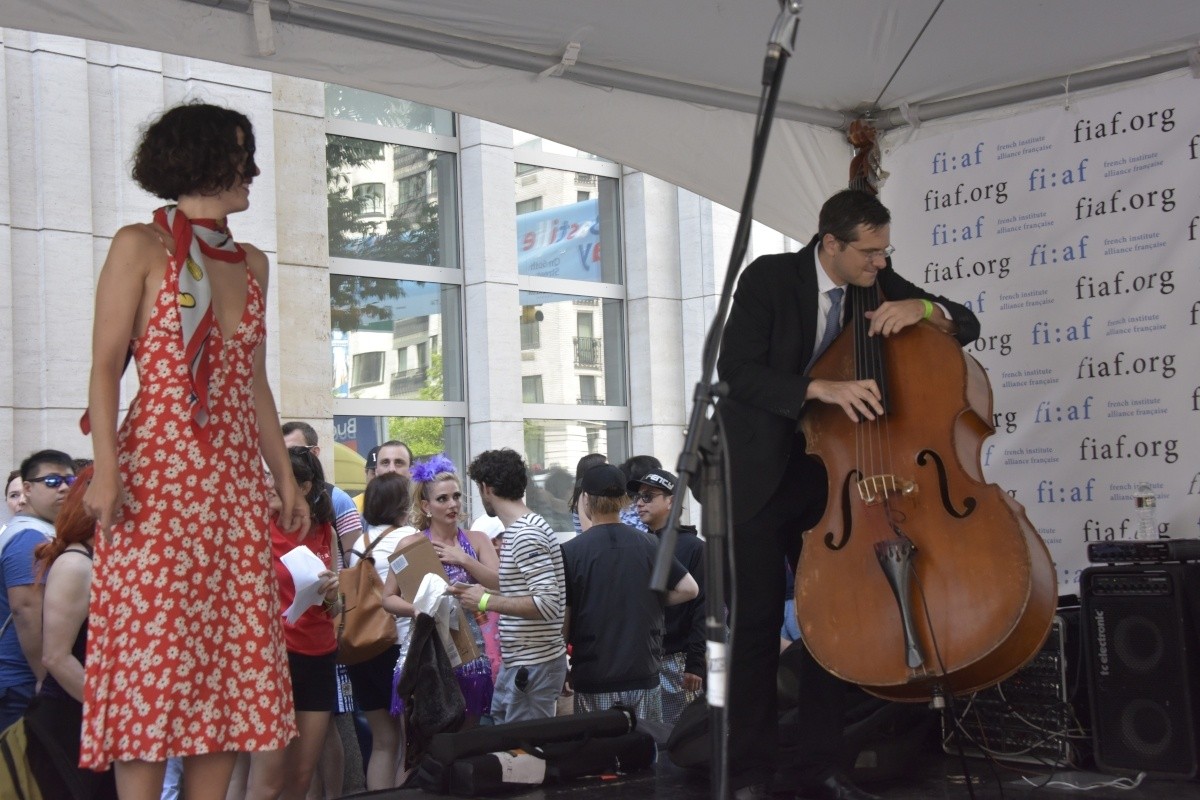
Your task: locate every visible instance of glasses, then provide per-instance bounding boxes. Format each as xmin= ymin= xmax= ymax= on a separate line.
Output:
xmin=838 ymin=238 xmax=896 ymax=259
xmin=27 ymin=474 xmax=79 ymax=488
xmin=631 ymin=492 xmax=671 ymax=503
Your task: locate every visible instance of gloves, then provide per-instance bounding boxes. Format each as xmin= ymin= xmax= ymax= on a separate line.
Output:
xmin=581 ymin=463 xmax=628 ymax=497
xmin=626 ymin=469 xmax=679 ymax=498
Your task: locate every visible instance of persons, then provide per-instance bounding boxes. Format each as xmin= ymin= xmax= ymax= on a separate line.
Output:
xmin=677 ymin=191 xmax=983 ymax=800
xmin=77 ymin=100 xmax=314 ymax=800
xmin=0 ymin=449 xmax=182 ymax=800
xmin=226 ymin=421 xmax=803 ymax=800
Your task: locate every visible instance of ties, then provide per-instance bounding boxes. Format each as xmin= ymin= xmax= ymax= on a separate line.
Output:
xmin=800 ymin=288 xmax=844 ymax=376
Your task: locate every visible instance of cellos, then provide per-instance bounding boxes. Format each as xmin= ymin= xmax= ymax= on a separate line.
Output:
xmin=795 ymin=121 xmax=1058 ymax=800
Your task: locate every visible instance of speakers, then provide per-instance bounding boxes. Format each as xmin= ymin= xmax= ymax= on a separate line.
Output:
xmin=1079 ymin=561 xmax=1200 ymax=778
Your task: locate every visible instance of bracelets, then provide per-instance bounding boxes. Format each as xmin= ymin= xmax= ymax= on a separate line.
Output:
xmin=922 ymin=298 xmax=935 ymax=318
xmin=324 ymin=593 xmax=339 ymax=606
xmin=479 ymin=593 xmax=491 ymax=611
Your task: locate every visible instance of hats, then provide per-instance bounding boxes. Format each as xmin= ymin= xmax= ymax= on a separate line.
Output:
xmin=366 ymin=446 xmax=381 ymax=468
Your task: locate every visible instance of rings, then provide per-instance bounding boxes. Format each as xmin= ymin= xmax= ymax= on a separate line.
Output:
xmin=439 ymin=552 xmax=441 ymax=556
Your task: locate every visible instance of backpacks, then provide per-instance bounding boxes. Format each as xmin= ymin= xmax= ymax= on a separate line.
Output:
xmin=334 ymin=525 xmax=406 ymax=665
